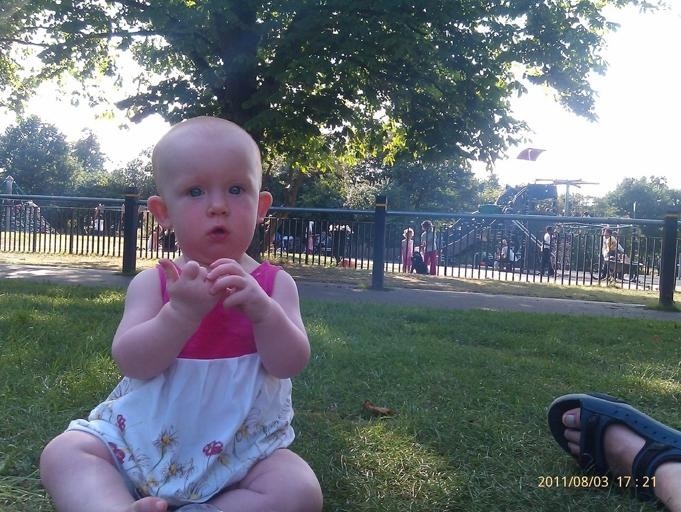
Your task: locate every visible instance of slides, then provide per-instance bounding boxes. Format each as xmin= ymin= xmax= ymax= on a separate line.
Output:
xmin=438 ymin=184 xmax=557 ymax=257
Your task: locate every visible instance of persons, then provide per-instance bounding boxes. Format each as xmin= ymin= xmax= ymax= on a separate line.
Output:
xmin=401 ymin=228 xmax=414 ymax=273
xmin=40 ymin=115 xmax=325 ymax=512
xmin=582 ymin=211 xmax=591 ymax=218
xmin=494 ymin=226 xmax=625 ymax=280
xmin=420 ymin=220 xmax=437 ymax=275
xmin=147 ymin=221 xmax=163 ymax=251
xmin=259 ymin=219 xmax=352 ymax=263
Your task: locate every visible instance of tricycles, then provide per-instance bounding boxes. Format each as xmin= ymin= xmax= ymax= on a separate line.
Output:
xmin=590 ymin=249 xmax=644 ymax=282
